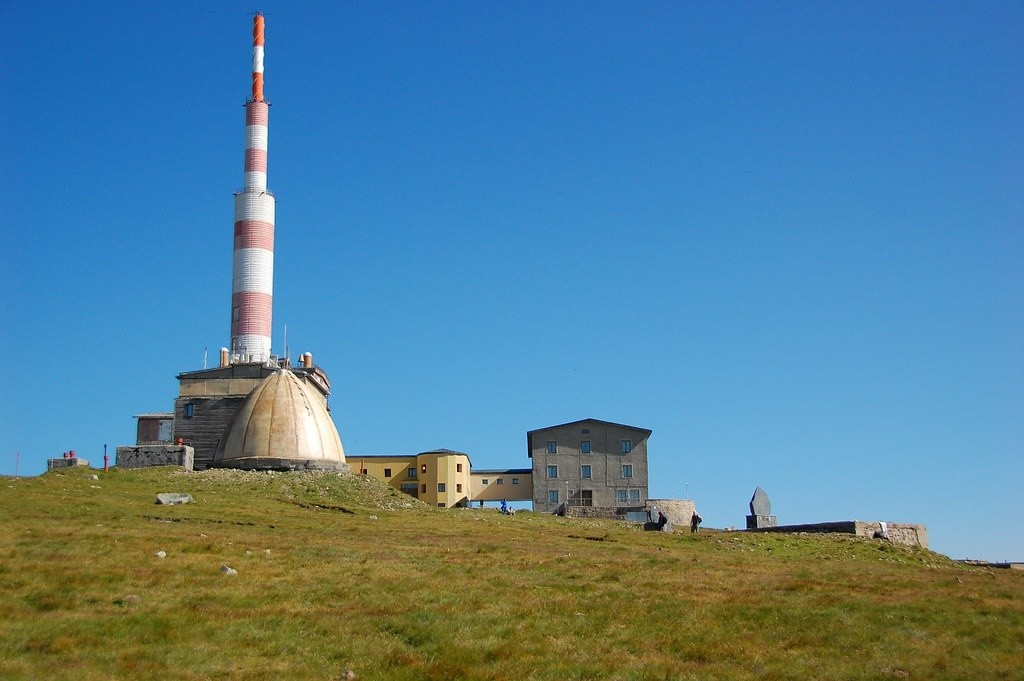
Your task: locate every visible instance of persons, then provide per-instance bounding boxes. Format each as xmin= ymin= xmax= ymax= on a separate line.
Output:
xmin=657 ymin=511 xmax=668 ymax=531
xmin=690 ymin=511 xmax=703 ymax=533
xmin=500 ymin=497 xmax=508 ymax=515
xmin=507 ymin=507 xmax=515 ymax=516
